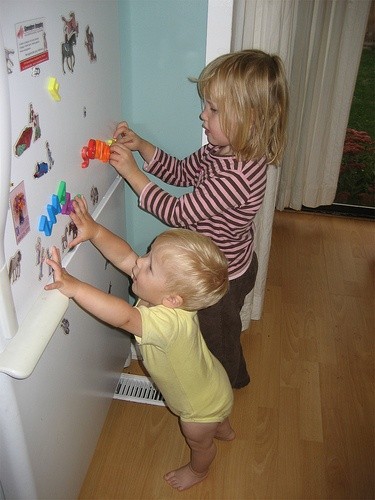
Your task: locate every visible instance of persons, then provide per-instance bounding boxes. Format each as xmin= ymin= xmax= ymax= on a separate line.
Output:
xmin=105 ymin=49 xmax=288 ymax=388
xmin=46 ymin=195 xmax=236 ymax=494
